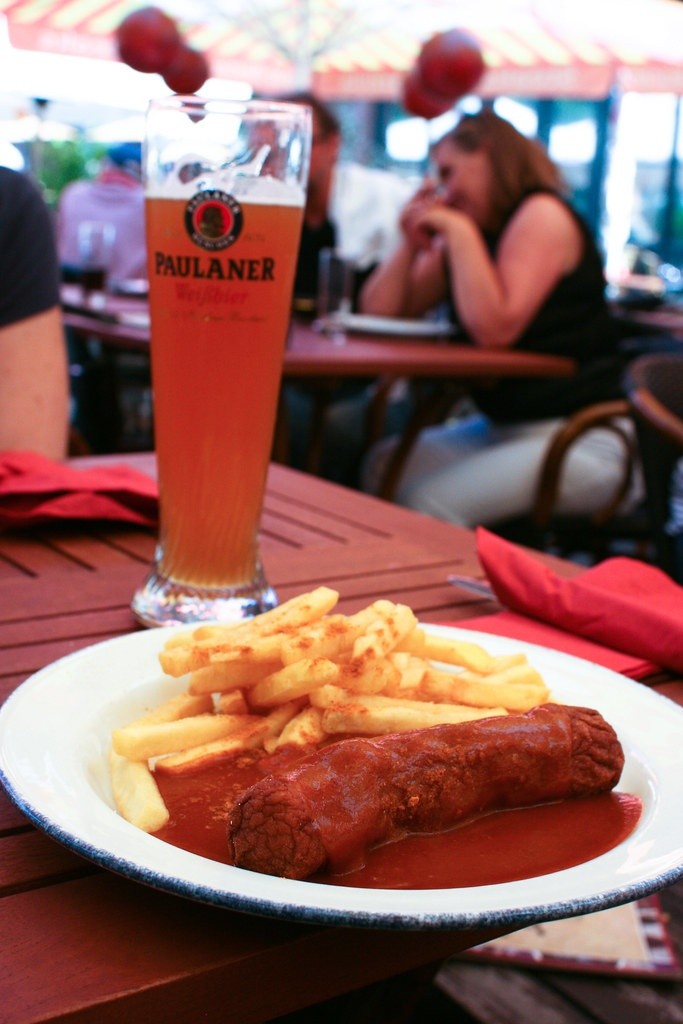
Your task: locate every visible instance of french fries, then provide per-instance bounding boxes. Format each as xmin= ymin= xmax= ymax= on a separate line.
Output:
xmin=109 ymin=585 xmax=557 ymax=834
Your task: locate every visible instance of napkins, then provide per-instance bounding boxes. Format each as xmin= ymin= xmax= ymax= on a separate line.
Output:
xmin=464 ymin=521 xmax=682 ymax=699
xmin=0 ymin=451 xmax=165 ymax=532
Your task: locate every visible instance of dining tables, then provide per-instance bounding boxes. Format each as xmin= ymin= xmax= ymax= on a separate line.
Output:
xmin=0 ymin=383 xmax=683 ymax=1023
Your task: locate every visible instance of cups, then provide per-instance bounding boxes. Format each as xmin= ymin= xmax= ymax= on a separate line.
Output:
xmin=130 ymin=96 xmax=312 ymax=629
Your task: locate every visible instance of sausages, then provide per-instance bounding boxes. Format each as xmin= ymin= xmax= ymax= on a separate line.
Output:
xmin=227 ymin=706 xmax=624 ymax=883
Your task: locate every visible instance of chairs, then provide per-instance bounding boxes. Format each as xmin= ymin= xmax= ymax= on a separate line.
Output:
xmin=515 ymin=351 xmax=683 ymax=591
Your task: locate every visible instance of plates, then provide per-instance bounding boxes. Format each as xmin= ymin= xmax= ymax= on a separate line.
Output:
xmin=1 ymin=622 xmax=683 ymax=924
xmin=333 ymin=311 xmax=449 ymax=334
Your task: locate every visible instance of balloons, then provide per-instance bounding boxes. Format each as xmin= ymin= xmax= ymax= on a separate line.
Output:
xmin=405 ymin=31 xmax=482 ymax=115
xmin=121 ymin=8 xmax=205 ymax=95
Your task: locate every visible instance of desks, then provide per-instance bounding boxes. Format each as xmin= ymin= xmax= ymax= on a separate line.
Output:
xmin=53 ymin=295 xmax=580 ymax=505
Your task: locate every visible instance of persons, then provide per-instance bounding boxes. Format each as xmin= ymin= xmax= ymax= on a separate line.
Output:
xmin=362 ymin=113 xmax=635 ymax=525
xmin=0 ymin=165 xmax=70 ymax=464
xmin=250 ymin=93 xmax=426 ymax=476
xmin=57 ymin=142 xmax=150 ymax=287
